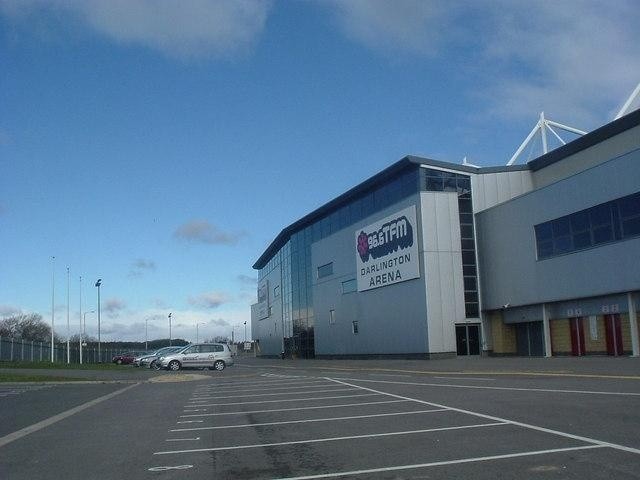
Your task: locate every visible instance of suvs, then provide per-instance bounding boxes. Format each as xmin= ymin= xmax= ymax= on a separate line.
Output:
xmin=112 ymin=343 xmax=234 ymax=371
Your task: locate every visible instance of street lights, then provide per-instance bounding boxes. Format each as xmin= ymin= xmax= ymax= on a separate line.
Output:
xmin=243 ymin=321 xmax=248 ymax=342
xmin=94 ymin=278 xmax=102 ymax=364
xmin=167 ymin=312 xmax=173 ymax=347
